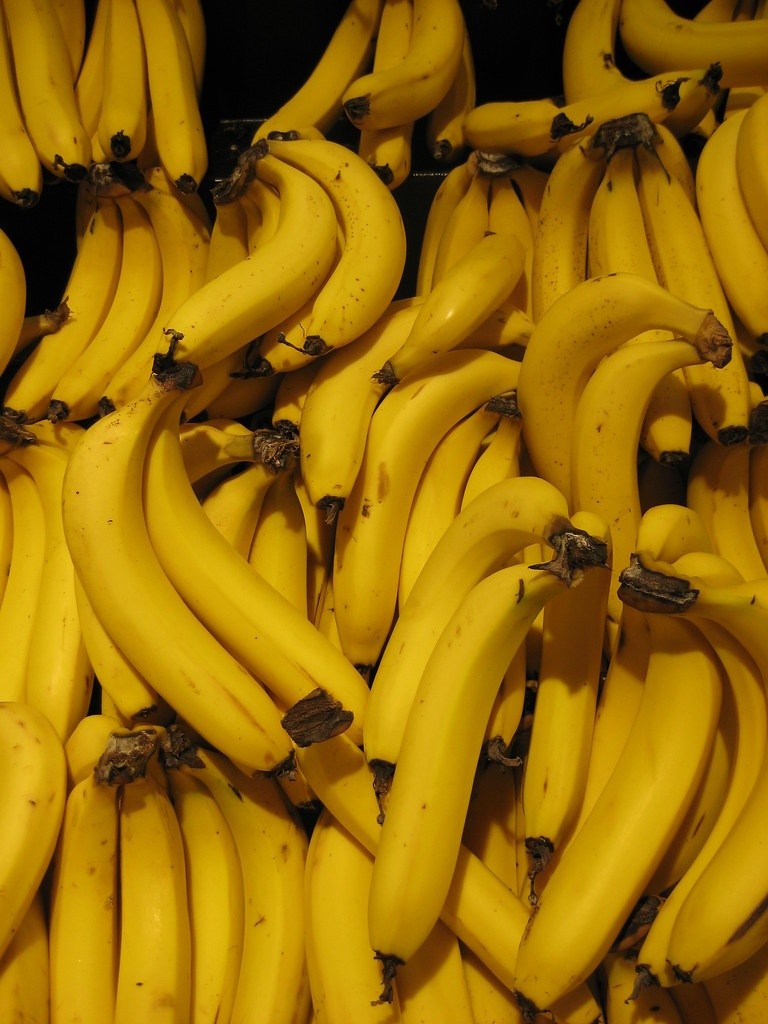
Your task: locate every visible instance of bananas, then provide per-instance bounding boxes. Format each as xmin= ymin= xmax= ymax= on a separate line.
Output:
xmin=0 ymin=0 xmax=768 ymax=1024
